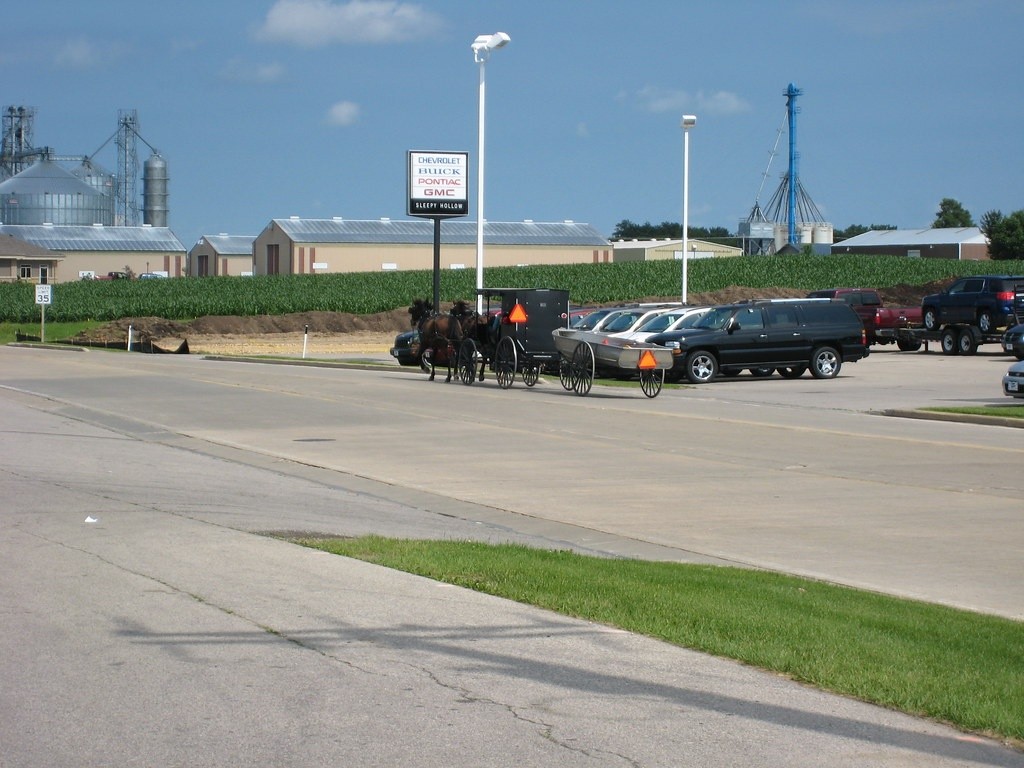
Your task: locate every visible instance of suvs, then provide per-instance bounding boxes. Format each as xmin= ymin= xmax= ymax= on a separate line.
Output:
xmin=921 ymin=273 xmax=1024 ymax=329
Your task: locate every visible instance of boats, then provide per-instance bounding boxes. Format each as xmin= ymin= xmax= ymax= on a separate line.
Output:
xmin=551 ymin=325 xmax=674 ymax=372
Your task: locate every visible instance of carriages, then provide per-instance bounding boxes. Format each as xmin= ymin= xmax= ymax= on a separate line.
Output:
xmin=407 ymin=285 xmax=570 ymax=387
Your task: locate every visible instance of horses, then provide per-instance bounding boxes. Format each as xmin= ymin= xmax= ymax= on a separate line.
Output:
xmin=408 ymin=297 xmax=509 ymax=383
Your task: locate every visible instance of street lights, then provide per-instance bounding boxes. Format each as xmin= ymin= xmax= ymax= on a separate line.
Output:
xmin=680 ymin=113 xmax=697 ymax=305
xmin=470 ymin=32 xmax=510 ymax=314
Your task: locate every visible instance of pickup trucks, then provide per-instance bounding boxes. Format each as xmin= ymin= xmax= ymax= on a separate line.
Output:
xmin=95 ymin=272 xmax=127 ymax=281
xmin=646 ymin=297 xmax=870 ymax=381
xmin=807 ymin=289 xmax=927 ymax=351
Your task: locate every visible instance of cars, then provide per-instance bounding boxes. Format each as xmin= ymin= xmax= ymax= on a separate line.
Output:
xmin=570 ymin=297 xmax=709 ymax=378
xmin=139 ymin=273 xmax=161 ymax=278
xmin=1001 ymin=323 xmax=1024 ymax=355
xmin=390 ymin=327 xmax=485 ymax=367
xmin=1002 ymin=362 xmax=1024 ymax=398
